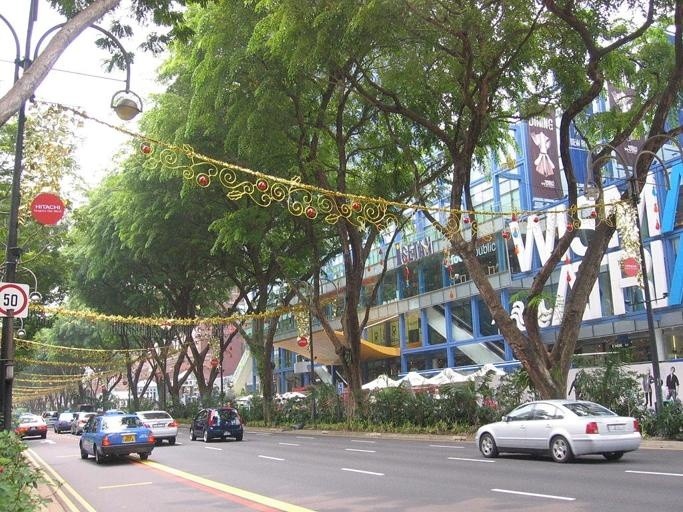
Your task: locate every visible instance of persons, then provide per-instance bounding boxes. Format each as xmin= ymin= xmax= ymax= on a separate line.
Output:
xmin=665 ymin=367 xmax=679 ymax=403
xmin=568 ymin=373 xmax=583 ymax=400
xmin=276 ymin=394 xmax=307 ymax=420
xmin=478 ymin=370 xmax=499 ymax=409
xmin=637 ymin=368 xmax=654 ymax=408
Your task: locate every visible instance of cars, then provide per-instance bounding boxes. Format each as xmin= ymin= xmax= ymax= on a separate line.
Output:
xmin=132 ymin=410 xmax=178 ymax=446
xmin=188 ymin=406 xmax=243 ymax=443
xmin=474 ymin=399 xmax=642 ymax=463
xmin=10 ymin=403 xmax=125 ymax=440
xmin=78 ymin=409 xmax=155 ymax=463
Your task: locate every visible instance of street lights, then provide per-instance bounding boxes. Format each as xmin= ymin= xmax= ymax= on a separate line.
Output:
xmin=583 ymin=134 xmax=682 ymax=438
xmin=0 ymin=255 xmax=43 ymax=339
xmin=45 ymin=367 xmax=175 ymax=411
xmin=277 ymin=277 xmax=340 ymax=424
xmin=194 ymin=308 xmax=247 ymax=407
xmin=0 ymin=1 xmax=143 ymax=446
